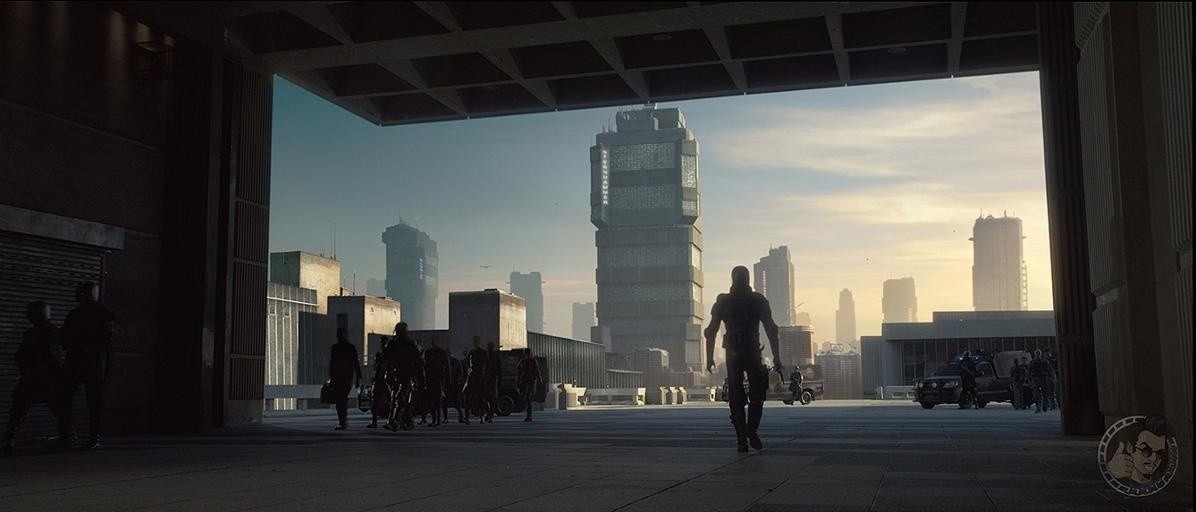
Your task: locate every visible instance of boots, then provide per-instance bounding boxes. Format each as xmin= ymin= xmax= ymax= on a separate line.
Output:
xmin=1035 ymin=403 xmax=1056 ymax=413
xmin=480 ymin=409 xmax=494 ymax=424
xmin=733 ymin=404 xmax=762 ymax=451
xmin=367 ymin=407 xmax=448 ymax=432
xmin=458 ymin=408 xmax=471 ymax=424
xmin=335 ymin=409 xmax=347 ymax=429
xmin=524 ymin=407 xmax=531 ymax=422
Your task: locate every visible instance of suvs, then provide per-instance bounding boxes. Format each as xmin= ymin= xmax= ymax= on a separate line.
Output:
xmin=352 ymin=346 xmax=550 ymax=424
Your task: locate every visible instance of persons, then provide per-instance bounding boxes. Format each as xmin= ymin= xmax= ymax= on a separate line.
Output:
xmin=788 ymin=365 xmax=805 ymax=405
xmin=52 ymin=280 xmax=120 ymax=450
xmin=948 ymin=349 xmax=983 ymax=410
xmin=1 ymin=299 xmax=64 ymax=451
xmin=363 ymin=321 xmax=539 ymax=431
xmin=705 ymin=264 xmax=783 ymax=453
xmin=1010 ymin=346 xmax=1060 ymax=413
xmin=1107 ymin=415 xmax=1171 ymax=487
xmin=319 ymin=325 xmax=361 ymax=430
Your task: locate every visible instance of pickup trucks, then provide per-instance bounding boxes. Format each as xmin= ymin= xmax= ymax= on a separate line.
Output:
xmin=910 ymin=350 xmax=1043 ymax=410
xmin=720 ymin=366 xmax=825 ymax=407
